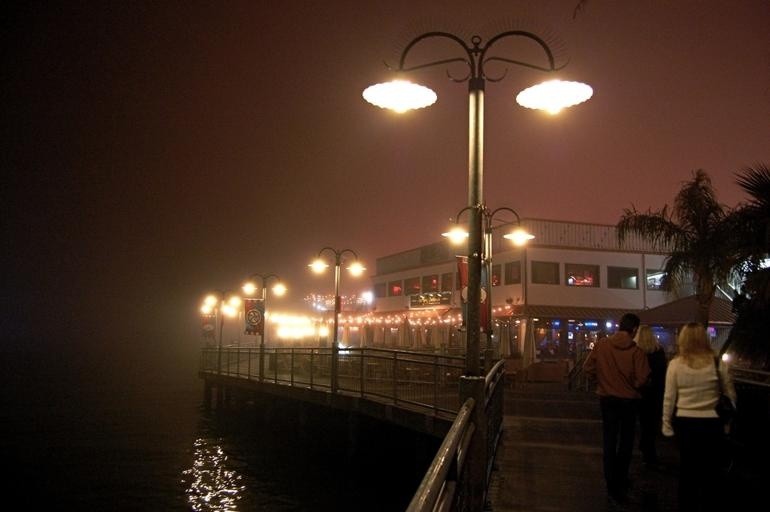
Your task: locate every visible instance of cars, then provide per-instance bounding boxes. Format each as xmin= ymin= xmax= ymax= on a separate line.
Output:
xmin=720 ymin=337 xmax=770 ymax=423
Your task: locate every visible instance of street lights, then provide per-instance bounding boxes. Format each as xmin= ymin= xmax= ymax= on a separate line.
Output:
xmin=242 ymin=272 xmax=286 ymax=383
xmin=360 ymin=30 xmax=595 ymax=397
xmin=442 ymin=202 xmax=537 ymax=376
xmin=307 ymin=245 xmax=368 ymax=397
xmin=198 ymin=291 xmax=242 ymax=375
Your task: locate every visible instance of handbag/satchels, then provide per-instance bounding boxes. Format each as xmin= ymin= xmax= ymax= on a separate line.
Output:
xmin=712 ymin=396 xmax=735 ymax=421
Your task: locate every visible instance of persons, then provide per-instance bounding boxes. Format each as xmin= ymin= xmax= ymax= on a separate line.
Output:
xmin=579 ymin=313 xmax=651 ymax=503
xmin=634 ymin=325 xmax=667 ymax=461
xmin=661 ymin=322 xmax=736 ymax=512
